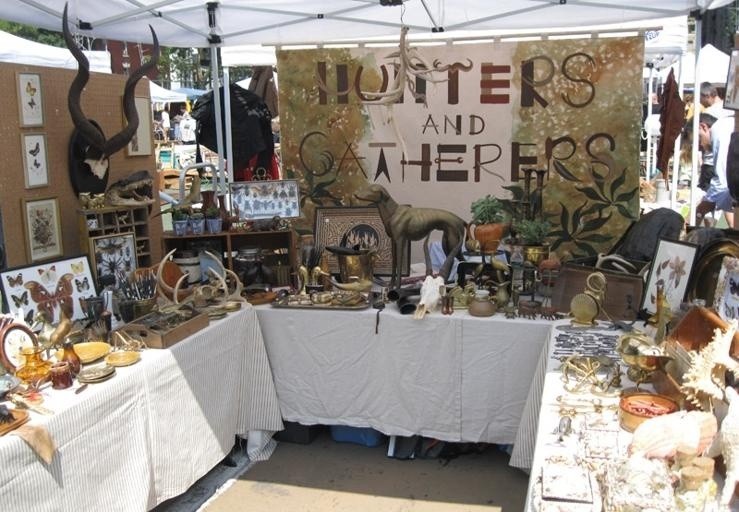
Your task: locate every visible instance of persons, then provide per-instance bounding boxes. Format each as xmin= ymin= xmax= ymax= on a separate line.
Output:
xmin=699 ymin=82 xmax=735 ymax=118
xmin=157 ymin=107 xmax=170 ymax=143
xmin=179 ymin=105 xmax=190 ymax=121
xmin=684 ymin=94 xmax=706 ymax=121
xmin=683 ymin=113 xmax=735 ymax=228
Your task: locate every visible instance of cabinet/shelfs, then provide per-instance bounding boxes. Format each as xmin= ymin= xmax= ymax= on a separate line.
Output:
xmin=75 ymin=205 xmax=154 ymax=269
xmin=161 ymin=230 xmax=298 ymax=294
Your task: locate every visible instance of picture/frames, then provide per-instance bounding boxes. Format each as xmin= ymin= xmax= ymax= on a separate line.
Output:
xmin=229 ymin=180 xmax=301 ymax=221
xmin=639 ymin=235 xmax=739 ymax=327
xmin=313 ymin=204 xmax=411 ymax=276
xmin=0 ymin=232 xmax=138 ymax=333
xmin=14 ymin=71 xmax=63 ymax=264
xmin=122 ymin=96 xmax=155 ymax=157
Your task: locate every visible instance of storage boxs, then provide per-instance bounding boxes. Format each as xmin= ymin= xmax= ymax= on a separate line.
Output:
xmin=272 ymin=421 xmax=321 ymax=445
xmin=330 ymin=424 xmax=388 ymax=447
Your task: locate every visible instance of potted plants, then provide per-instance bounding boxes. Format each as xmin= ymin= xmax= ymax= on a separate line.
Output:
xmin=170 ymin=207 xmax=223 ymax=237
xmin=471 ymin=194 xmax=550 ymax=267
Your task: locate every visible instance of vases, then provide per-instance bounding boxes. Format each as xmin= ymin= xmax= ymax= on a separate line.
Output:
xmin=201 ymin=191 xmax=229 ymax=230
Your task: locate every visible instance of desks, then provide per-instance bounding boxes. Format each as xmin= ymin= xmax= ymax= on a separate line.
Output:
xmin=1 ymin=285 xmax=286 ymax=511
xmin=250 ymin=286 xmax=568 ymax=460
xmin=509 ymin=317 xmax=738 ymax=510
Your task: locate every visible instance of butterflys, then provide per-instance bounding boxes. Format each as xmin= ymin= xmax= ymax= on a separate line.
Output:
xmin=25 ymin=80 xmax=37 ymax=108
xmin=28 ymin=142 xmax=41 ymax=169
xmin=6 ymin=260 xmax=94 ymax=329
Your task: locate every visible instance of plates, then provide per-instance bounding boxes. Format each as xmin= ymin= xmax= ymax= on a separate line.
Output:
xmin=53 ymin=341 xmax=140 ymax=384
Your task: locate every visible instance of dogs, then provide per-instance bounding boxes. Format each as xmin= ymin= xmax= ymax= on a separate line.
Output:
xmin=354 ymin=183 xmax=471 ymax=290
